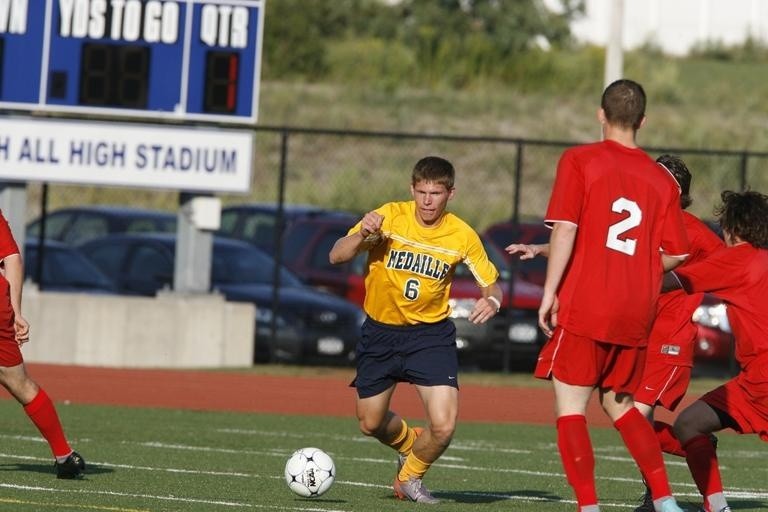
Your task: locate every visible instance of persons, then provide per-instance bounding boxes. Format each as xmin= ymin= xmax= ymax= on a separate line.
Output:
xmin=505 ymin=155 xmax=724 ymax=512
xmin=328 ymin=156 xmax=504 ymax=505
xmin=654 ymin=186 xmax=768 ymax=511
xmin=533 ymin=79 xmax=692 ymax=512
xmin=0 ymin=210 xmax=86 ymax=478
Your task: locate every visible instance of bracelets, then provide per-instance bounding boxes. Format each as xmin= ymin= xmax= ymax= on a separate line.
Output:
xmin=488 ymin=295 xmax=502 ymax=314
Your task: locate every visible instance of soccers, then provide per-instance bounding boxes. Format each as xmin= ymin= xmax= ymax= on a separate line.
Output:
xmin=283 ymin=446 xmax=337 ymax=498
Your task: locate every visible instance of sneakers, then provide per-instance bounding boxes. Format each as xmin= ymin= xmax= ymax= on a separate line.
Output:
xmin=53 ymin=451 xmax=86 ymax=481
xmin=633 ymin=485 xmax=656 ymax=512
xmin=394 ymin=474 xmax=441 ymax=504
xmin=708 ymin=432 xmax=718 ymax=452
xmin=393 ymin=427 xmax=425 ymax=500
